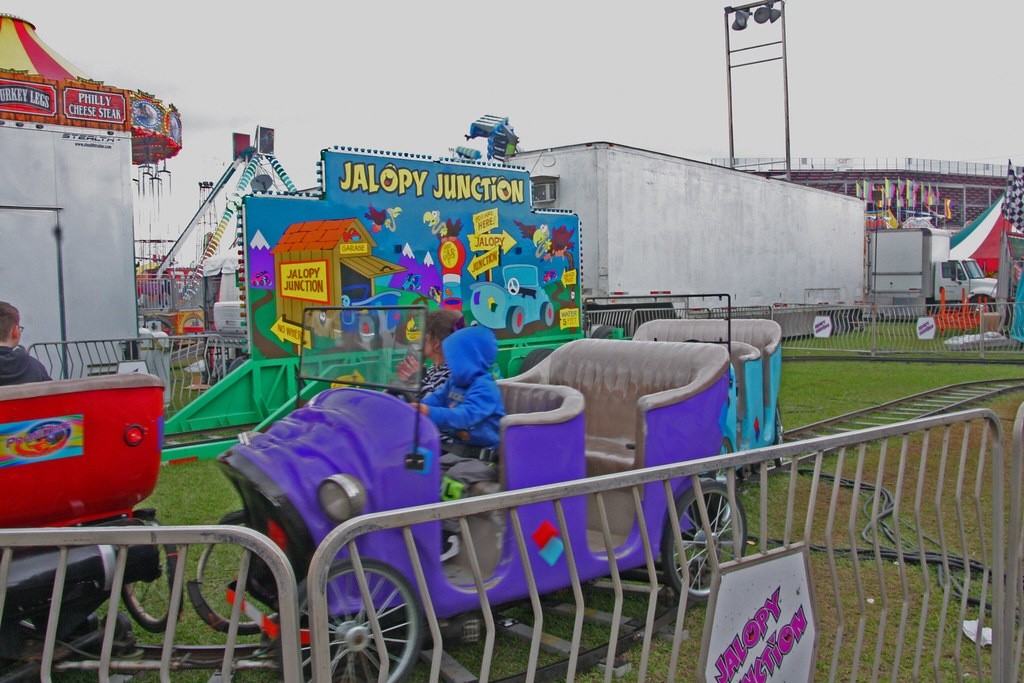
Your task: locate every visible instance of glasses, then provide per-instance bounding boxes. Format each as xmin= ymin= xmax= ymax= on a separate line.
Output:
xmin=19 ymin=326 xmax=23 ymax=332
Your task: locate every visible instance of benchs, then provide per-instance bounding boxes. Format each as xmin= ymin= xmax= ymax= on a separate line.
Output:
xmin=585 ymin=436 xmax=640 ymax=493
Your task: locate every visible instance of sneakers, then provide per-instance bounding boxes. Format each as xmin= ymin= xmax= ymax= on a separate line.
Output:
xmin=440 ymin=538 xmax=461 ymax=564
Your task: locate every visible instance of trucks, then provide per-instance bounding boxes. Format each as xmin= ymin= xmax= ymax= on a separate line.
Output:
xmin=869 ymin=228 xmax=1014 ymax=326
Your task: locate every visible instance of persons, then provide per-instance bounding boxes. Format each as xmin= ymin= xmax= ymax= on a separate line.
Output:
xmin=407 ymin=326 xmax=507 ymax=563
xmin=397 ymin=308 xmax=468 ymax=459
xmin=0 ymin=301 xmax=53 ymax=387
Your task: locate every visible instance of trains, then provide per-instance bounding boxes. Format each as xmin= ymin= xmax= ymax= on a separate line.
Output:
xmin=0 ymin=286 xmax=788 ymax=683
